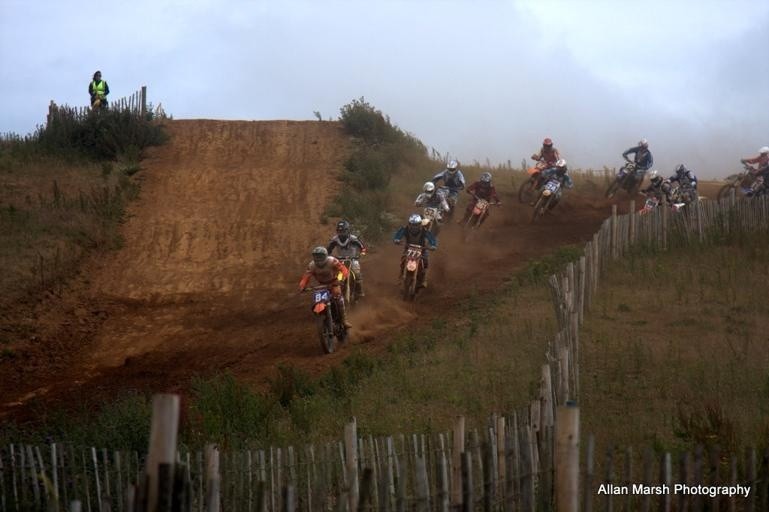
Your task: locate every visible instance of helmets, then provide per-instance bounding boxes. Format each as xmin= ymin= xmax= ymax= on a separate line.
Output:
xmin=676 ymin=163 xmax=687 ymax=175
xmin=648 ymin=170 xmax=662 ymax=186
xmin=639 ymin=138 xmax=648 ymax=149
xmin=480 ymin=172 xmax=492 ymax=182
xmin=408 ymin=214 xmax=422 ymax=232
xmin=543 ymin=137 xmax=553 ymax=145
xmin=556 ymin=159 xmax=567 ymax=175
xmin=423 ymin=182 xmax=435 ymax=200
xmin=759 ymin=147 xmax=769 ymax=153
xmin=312 ymin=247 xmax=329 ymax=268
xmin=337 ymin=221 xmax=349 ymax=238
xmin=447 ymin=161 xmax=458 ymax=174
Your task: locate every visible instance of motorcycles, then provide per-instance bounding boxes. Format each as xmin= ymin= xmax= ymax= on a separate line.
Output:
xmin=332 ymin=253 xmax=366 ymax=304
xmin=433 ymin=182 xmax=461 ymax=221
xmin=300 ymin=277 xmax=348 ymax=357
xmin=415 ymin=203 xmax=444 ymax=234
xmin=603 ymin=150 xmax=769 ymax=225
xmin=396 ymin=241 xmax=432 ymax=303
xmin=517 ymin=155 xmax=553 ymax=203
xmin=527 ymin=174 xmax=567 ymax=223
xmin=461 ymin=189 xmax=496 ymax=239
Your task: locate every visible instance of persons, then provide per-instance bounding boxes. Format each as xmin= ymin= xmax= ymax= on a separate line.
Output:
xmin=530 ymin=158 xmax=572 ymax=216
xmin=415 ymin=182 xmax=450 ymax=236
xmin=89 ymin=70 xmax=109 ymax=114
xmin=456 ymin=172 xmax=503 ymax=231
xmin=530 ymin=138 xmax=560 ymax=167
xmin=431 ymin=161 xmax=465 ymax=214
xmin=327 ymin=220 xmax=366 ymax=297
xmin=623 ymin=139 xmax=769 ymax=207
xmin=297 ymin=247 xmax=352 ymax=329
xmin=393 ymin=215 xmax=436 ymax=287
